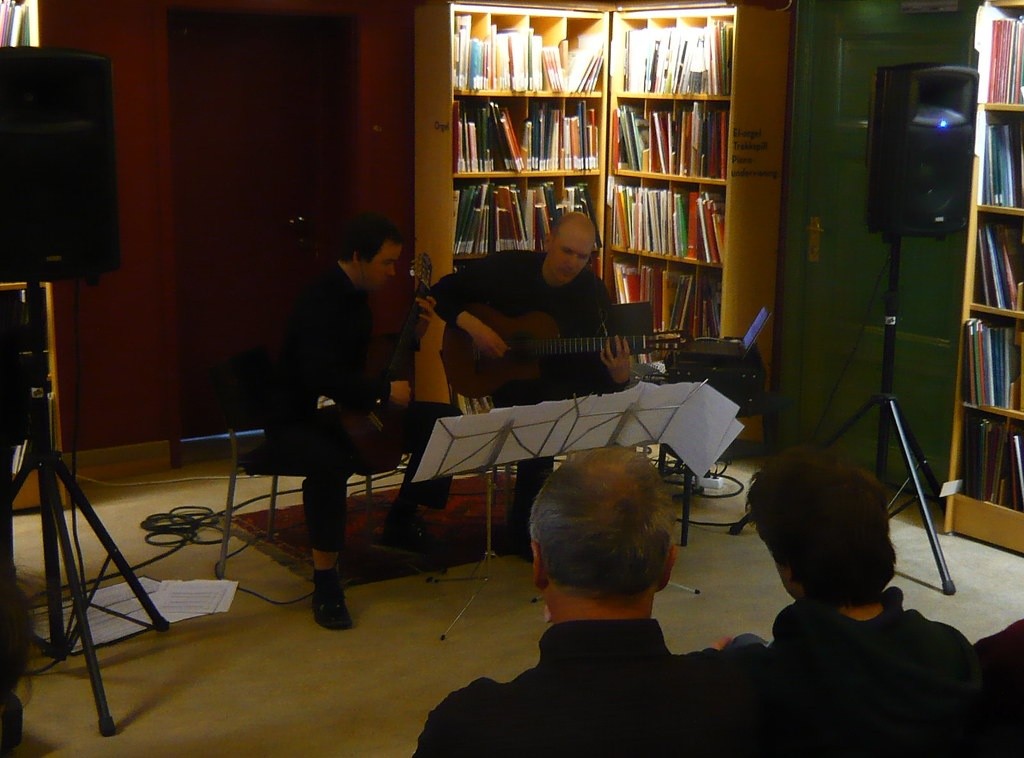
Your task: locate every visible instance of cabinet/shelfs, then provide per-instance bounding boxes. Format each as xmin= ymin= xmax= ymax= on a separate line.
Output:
xmin=414 ymin=2 xmax=791 ymax=442
xmin=0 ymin=0 xmax=72 ymax=511
xmin=943 ymin=0 xmax=1024 ymax=558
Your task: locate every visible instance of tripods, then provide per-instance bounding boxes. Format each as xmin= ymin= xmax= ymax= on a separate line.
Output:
xmin=2 ymin=282 xmax=171 ymax=738
xmin=730 ymin=240 xmax=956 ymax=595
xmin=413 ymin=391 xmax=594 ymax=640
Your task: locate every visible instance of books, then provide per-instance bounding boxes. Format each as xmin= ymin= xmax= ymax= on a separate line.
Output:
xmin=453 ymin=16 xmax=732 ymax=363
xmin=958 ymin=17 xmax=1024 ymax=515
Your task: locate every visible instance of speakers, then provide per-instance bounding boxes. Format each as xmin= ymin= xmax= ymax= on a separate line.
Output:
xmin=864 ymin=62 xmax=980 ymax=238
xmin=0 ymin=46 xmax=117 ymax=290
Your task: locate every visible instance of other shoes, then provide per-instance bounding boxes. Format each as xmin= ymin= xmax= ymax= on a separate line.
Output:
xmin=385 ymin=514 xmax=441 ymax=558
xmin=510 ymin=526 xmax=535 ymax=563
xmin=313 ymin=588 xmax=352 ymax=629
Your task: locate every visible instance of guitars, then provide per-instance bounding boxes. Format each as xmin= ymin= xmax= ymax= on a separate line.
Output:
xmin=332 ymin=246 xmax=435 ymax=475
xmin=440 ymin=301 xmax=693 ymax=400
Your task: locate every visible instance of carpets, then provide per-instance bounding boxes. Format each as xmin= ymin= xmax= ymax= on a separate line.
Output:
xmin=222 ymin=471 xmax=516 ymax=591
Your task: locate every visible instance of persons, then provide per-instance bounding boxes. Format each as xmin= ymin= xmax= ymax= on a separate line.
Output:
xmin=713 ymin=451 xmax=1024 ymax=758
xmin=429 ymin=215 xmax=631 ymax=564
xmin=409 ymin=446 xmax=782 ymax=758
xmin=975 ymin=619 xmax=1023 ymax=674
xmin=265 ymin=214 xmax=465 ymax=631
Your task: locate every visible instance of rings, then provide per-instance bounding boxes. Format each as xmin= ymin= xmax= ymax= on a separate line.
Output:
xmin=609 ymin=356 xmax=615 ymax=362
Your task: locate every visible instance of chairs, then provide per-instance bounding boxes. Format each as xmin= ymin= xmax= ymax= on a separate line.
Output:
xmin=211 ymin=346 xmax=371 ymax=562
xmin=439 ymin=320 xmax=573 ymax=524
